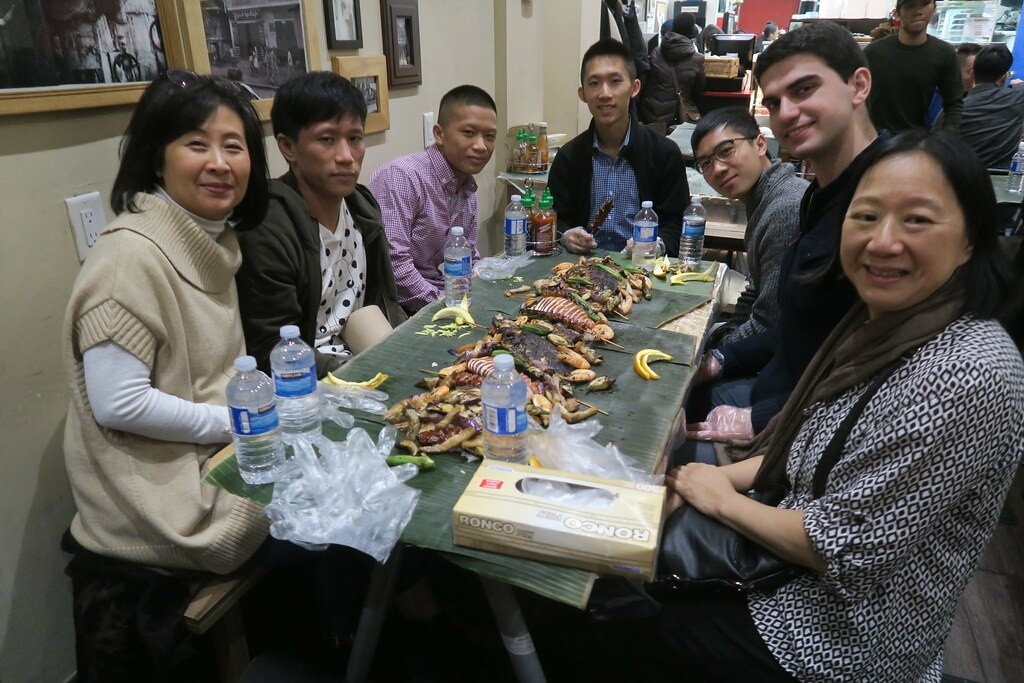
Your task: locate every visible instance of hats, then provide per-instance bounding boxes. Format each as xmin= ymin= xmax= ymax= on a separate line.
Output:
xmin=672 ymin=12 xmax=698 ymax=40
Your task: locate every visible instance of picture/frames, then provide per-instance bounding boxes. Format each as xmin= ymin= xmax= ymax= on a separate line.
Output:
xmin=379 ymin=0 xmax=422 ymax=91
xmin=322 ymin=0 xmax=364 ymax=50
xmin=0 ymin=0 xmax=323 ymax=123
xmin=331 ymin=56 xmax=390 ymax=135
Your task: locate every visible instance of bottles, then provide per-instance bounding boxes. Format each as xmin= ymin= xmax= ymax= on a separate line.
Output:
xmin=679 ymin=197 xmax=706 ymax=265
xmin=631 ymin=200 xmax=658 ymax=272
xmin=1004 ymin=142 xmax=1024 ymax=193
xmin=507 ymin=122 xmax=549 ymax=174
xmin=479 ymin=353 xmax=530 ymax=466
xmin=521 ymin=187 xmax=557 ymax=256
xmin=269 ymin=325 xmax=323 ymax=447
xmin=503 ymin=194 xmax=527 ymax=260
xmin=225 ymin=355 xmax=286 ymax=485
xmin=442 ymin=226 xmax=473 ymax=308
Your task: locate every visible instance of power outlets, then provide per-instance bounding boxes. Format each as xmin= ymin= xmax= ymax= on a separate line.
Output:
xmin=423 ymin=112 xmax=436 ymax=149
xmin=64 ymin=191 xmax=109 ymax=265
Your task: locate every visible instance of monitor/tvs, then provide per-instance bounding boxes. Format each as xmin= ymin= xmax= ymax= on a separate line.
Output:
xmin=711 ymin=33 xmax=756 ymax=77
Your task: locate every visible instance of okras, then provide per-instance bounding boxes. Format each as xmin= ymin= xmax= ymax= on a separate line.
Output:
xmin=491 ymin=310 xmax=552 ymax=368
xmin=386 ymin=454 xmax=435 ymax=470
xmin=565 ymin=263 xmax=652 ymax=322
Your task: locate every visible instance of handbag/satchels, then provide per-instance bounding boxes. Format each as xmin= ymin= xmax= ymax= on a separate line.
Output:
xmin=643 ymin=481 xmax=805 ymax=593
xmin=679 ymin=97 xmax=701 ymax=124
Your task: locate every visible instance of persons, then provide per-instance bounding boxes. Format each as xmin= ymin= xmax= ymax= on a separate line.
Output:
xmin=61 ymin=69 xmax=503 ymax=683
xmin=932 ymin=42 xmax=1024 ymax=170
xmin=666 ymin=20 xmax=891 ymax=479
xmin=248 ymin=46 xmax=298 ymax=81
xmin=357 ymin=79 xmax=370 ymax=89
xmin=861 ymin=0 xmax=963 ymax=138
xmin=53 ymin=34 xmax=140 ymax=82
xmin=235 ymin=71 xmax=412 ymax=380
xmin=636 ymin=12 xmax=727 ymax=133
xmin=514 ymin=125 xmax=1024 ymax=683
xmin=683 ymin=109 xmax=813 ymax=384
xmin=546 ymin=38 xmax=691 ymax=258
xmin=755 ymin=23 xmax=778 ymax=55
xmin=365 ymin=85 xmax=498 ymax=315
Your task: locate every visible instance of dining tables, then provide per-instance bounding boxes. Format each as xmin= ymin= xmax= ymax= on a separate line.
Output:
xmin=207 ymin=246 xmax=727 ymax=683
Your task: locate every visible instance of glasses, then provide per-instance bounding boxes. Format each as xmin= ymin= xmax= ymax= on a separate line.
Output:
xmin=149 ymin=69 xmax=242 ymax=95
xmin=694 ymin=138 xmax=755 ymax=174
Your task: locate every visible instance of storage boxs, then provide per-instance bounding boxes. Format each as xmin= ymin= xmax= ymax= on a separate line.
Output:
xmin=704 ymin=56 xmax=739 ymax=78
xmin=451 ymin=458 xmax=667 ymax=582
